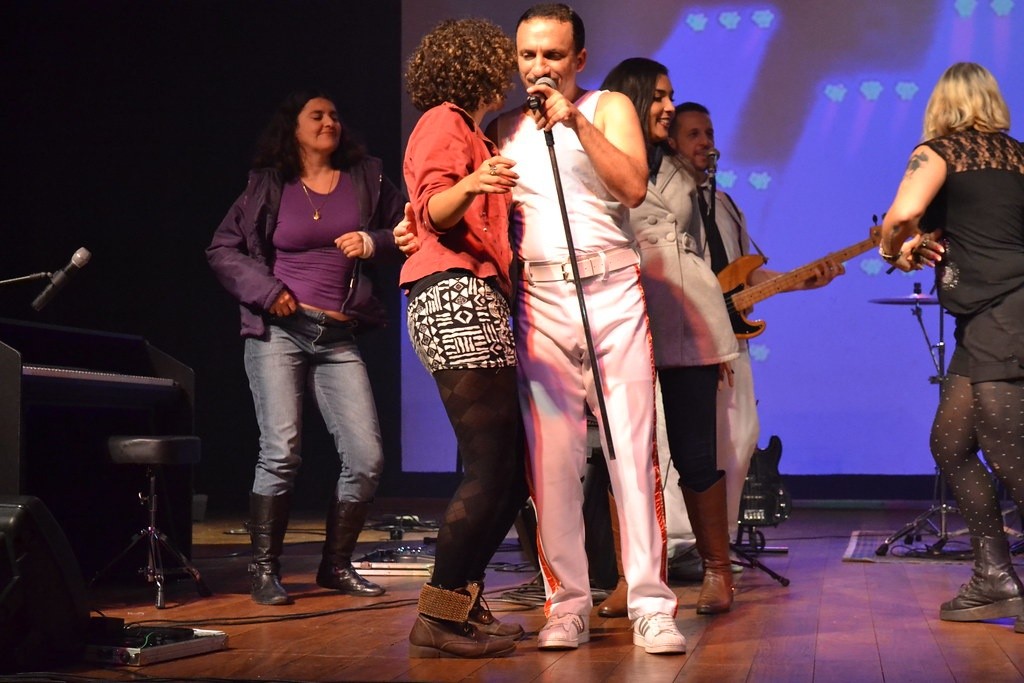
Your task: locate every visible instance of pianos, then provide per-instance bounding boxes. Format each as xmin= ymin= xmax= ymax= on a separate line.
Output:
xmin=0 ymin=317 xmax=197 ymax=589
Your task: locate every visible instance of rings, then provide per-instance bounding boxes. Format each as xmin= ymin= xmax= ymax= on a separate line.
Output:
xmin=920 ymin=234 xmax=930 ymax=247
xmin=490 ymin=166 xmax=497 ymax=175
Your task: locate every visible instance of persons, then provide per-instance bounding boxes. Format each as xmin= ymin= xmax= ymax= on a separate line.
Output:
xmin=878 ymin=62 xmax=1024 ymax=633
xmin=597 ymin=57 xmax=845 ymax=617
xmin=205 ymin=86 xmax=406 ymax=604
xmin=399 ymin=16 xmax=526 ymax=658
xmin=393 ymin=3 xmax=688 ymax=653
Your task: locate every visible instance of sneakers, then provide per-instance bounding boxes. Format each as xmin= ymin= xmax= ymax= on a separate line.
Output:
xmin=537 ymin=613 xmax=590 ymax=648
xmin=634 ymin=613 xmax=687 ymax=654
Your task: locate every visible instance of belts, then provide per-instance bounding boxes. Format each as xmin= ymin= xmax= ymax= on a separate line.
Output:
xmin=517 ymin=245 xmax=640 ymax=282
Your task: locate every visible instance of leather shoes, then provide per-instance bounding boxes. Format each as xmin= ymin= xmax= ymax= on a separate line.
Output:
xmin=407 ymin=582 xmax=526 ymax=659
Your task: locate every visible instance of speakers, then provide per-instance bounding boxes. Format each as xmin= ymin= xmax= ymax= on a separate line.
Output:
xmin=0 ymin=493 xmax=91 ymax=677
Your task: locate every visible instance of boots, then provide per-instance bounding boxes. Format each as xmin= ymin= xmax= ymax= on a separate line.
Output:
xmin=316 ymin=499 xmax=387 ymax=597
xmin=937 ymin=534 xmax=1024 ymax=622
xmin=1015 ymin=613 xmax=1024 ymax=633
xmin=597 ymin=490 xmax=628 ymax=619
xmin=244 ymin=490 xmax=291 ymax=605
xmin=678 ymin=469 xmax=734 ymax=613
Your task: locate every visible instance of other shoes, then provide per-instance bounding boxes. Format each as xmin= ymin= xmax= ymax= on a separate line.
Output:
xmin=668 ymin=549 xmax=743 ymax=580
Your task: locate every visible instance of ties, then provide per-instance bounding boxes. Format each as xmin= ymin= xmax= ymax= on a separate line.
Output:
xmin=696 ymin=186 xmax=729 ymax=276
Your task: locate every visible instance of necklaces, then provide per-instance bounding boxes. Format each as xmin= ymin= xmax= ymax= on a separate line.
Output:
xmin=299 ymin=169 xmax=335 ymax=220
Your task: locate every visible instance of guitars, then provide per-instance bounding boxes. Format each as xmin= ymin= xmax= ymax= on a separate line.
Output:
xmin=737 ymin=435 xmax=793 ymax=527
xmin=716 ymin=211 xmax=886 ymax=340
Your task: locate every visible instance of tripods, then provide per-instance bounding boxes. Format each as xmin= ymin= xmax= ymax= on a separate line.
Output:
xmin=874 ymin=304 xmax=1024 ymax=559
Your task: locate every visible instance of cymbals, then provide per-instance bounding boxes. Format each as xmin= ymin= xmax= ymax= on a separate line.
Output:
xmin=867 ymin=294 xmax=939 ymax=304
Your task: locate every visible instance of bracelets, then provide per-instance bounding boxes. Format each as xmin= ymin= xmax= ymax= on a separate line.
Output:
xmin=878 ymin=239 xmax=903 ymax=261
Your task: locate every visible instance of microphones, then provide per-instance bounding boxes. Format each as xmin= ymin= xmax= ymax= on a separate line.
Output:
xmin=30 ymin=248 xmax=92 ymax=311
xmin=527 ymin=77 xmax=557 ymax=110
xmin=705 ymin=148 xmax=721 ymax=173
